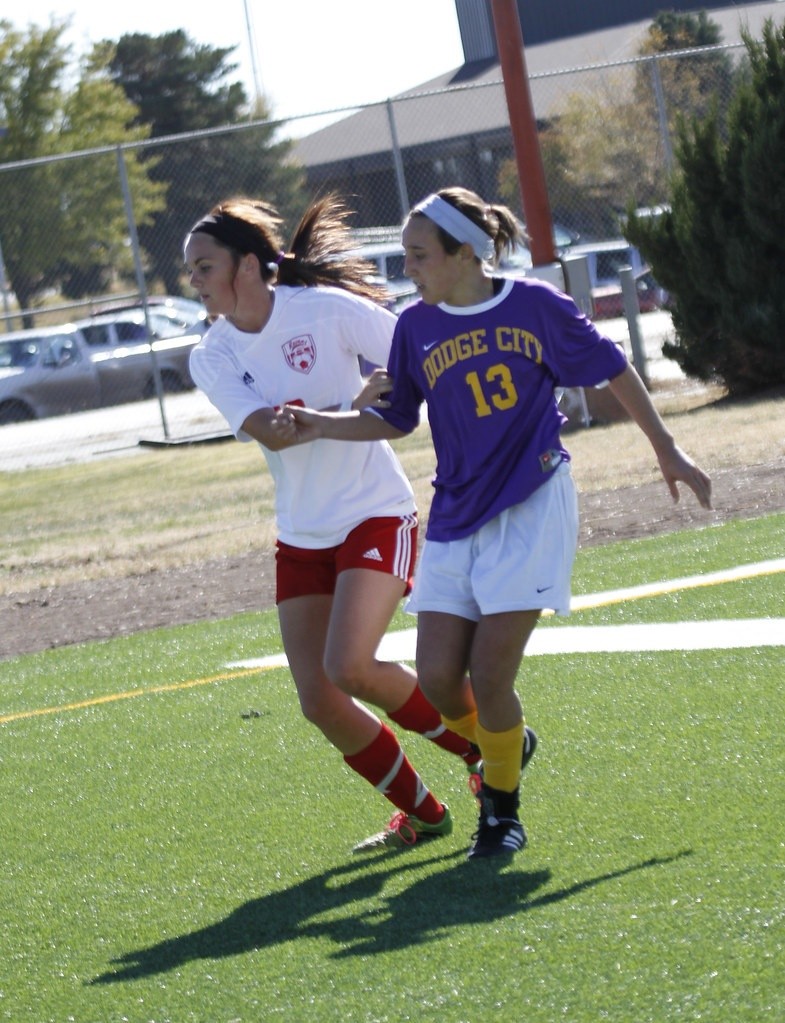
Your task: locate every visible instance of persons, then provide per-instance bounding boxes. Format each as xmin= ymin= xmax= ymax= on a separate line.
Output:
xmin=182 ymin=191 xmax=535 ymax=853
xmin=270 ymin=186 xmax=715 ymax=861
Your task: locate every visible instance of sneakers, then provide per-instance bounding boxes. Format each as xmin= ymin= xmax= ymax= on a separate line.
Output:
xmin=480 ymin=725 xmax=536 ymax=786
xmin=352 ymin=802 xmax=453 ymax=855
xmin=465 ymin=814 xmax=524 ymax=863
xmin=467 ymin=772 xmax=480 ymax=803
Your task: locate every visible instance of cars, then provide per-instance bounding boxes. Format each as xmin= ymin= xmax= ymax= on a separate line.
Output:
xmin=0 ymin=293 xmax=224 ymax=427
xmin=311 ymin=201 xmax=676 ymax=321
